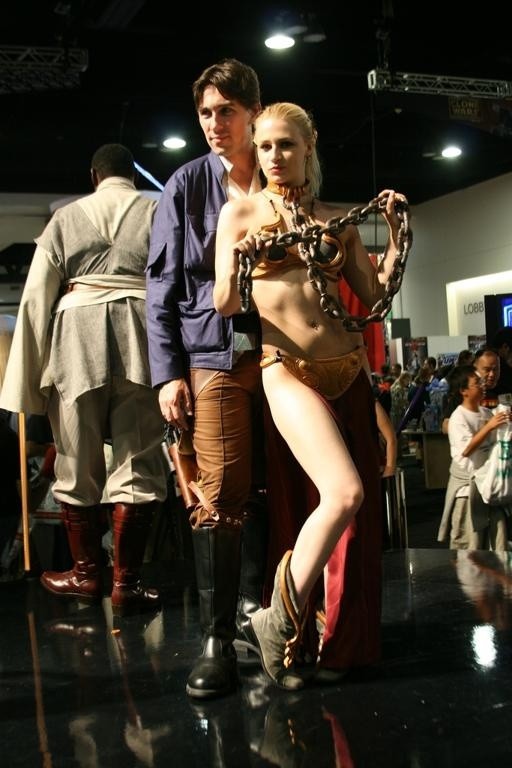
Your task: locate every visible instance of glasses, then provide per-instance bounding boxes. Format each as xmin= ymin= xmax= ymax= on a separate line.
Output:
xmin=470 ymin=378 xmax=486 ymax=389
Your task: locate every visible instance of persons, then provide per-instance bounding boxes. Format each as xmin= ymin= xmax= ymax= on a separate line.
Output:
xmin=0 ymin=143 xmax=164 ymax=618
xmin=456 ymin=548 xmax=512 ymax=630
xmin=143 ymin=56 xmax=267 ymax=698
xmin=370 ymin=326 xmax=511 ymax=549
xmin=210 ymin=100 xmax=413 ymax=694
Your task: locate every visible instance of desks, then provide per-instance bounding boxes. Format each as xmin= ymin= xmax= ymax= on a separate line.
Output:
xmin=402 ymin=424 xmax=454 ymax=489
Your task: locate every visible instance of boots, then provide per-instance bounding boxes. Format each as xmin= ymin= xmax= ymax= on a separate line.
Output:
xmin=104 ymin=596 xmax=160 ymax=731
xmin=233 ymin=505 xmax=272 ymax=670
xmin=42 ymin=502 xmax=105 ymax=599
xmin=249 ymin=549 xmax=310 ymax=691
xmin=44 ymin=604 xmax=111 ymax=678
xmin=184 ymin=527 xmax=241 ymax=700
xmin=238 ymin=662 xmax=273 ymax=754
xmin=188 ymin=696 xmax=250 ymax=767
xmin=301 ymin=600 xmax=327 ymax=664
xmin=256 ymin=694 xmax=310 ymax=768
xmin=110 ymin=503 xmax=153 ymax=608
